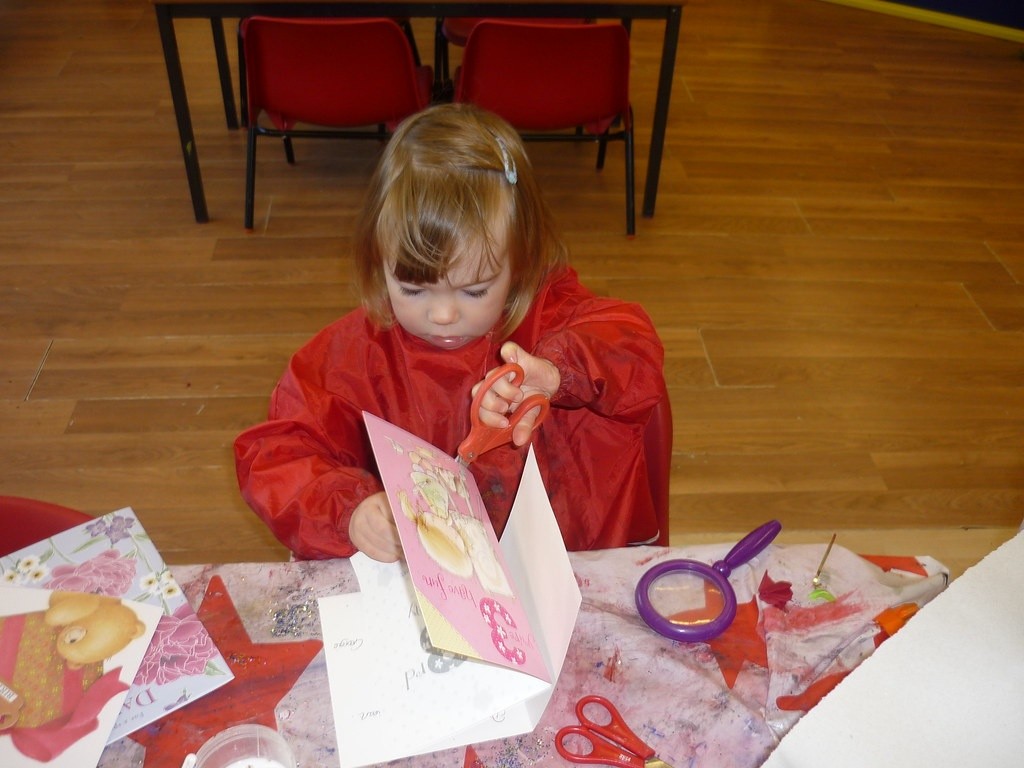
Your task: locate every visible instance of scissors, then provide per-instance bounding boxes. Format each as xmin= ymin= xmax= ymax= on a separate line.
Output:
xmin=453 ymin=363 xmax=550 ymax=469
xmin=552 ymin=693 xmax=670 ymax=768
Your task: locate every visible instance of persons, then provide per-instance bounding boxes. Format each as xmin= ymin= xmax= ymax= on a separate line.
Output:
xmin=235 ymin=105 xmax=675 ymax=560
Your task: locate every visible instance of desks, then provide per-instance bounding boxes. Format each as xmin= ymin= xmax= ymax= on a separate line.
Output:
xmin=154 ymin=0 xmax=685 ymax=223
xmin=98 ymin=547 xmax=957 ymax=766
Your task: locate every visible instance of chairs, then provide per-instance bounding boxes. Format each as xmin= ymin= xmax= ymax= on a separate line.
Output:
xmin=241 ymin=16 xmax=430 ymax=235
xmin=453 ymin=19 xmax=635 ymax=239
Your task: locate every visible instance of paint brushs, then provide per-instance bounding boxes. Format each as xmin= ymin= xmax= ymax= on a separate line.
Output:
xmin=810 ymin=532 xmax=838 ymax=591
